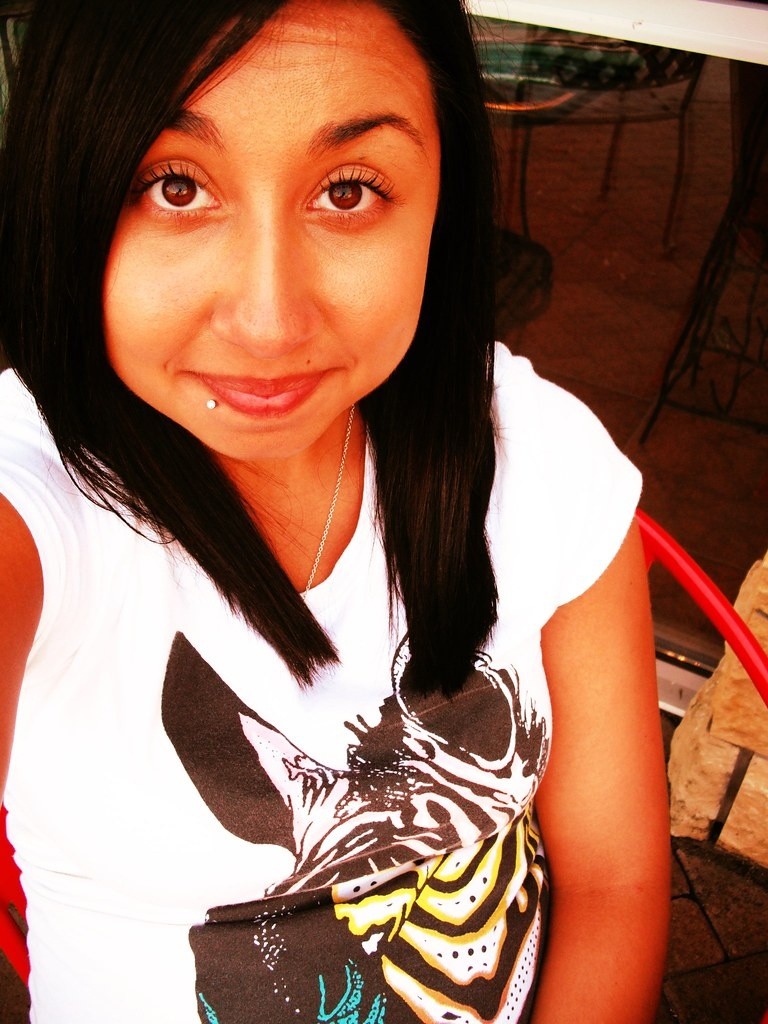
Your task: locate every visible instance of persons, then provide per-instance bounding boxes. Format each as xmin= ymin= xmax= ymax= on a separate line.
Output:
xmin=0 ymin=0 xmax=676 ymax=1022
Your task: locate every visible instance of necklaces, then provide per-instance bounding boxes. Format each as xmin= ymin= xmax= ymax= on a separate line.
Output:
xmin=301 ymin=399 xmax=359 ymax=601
